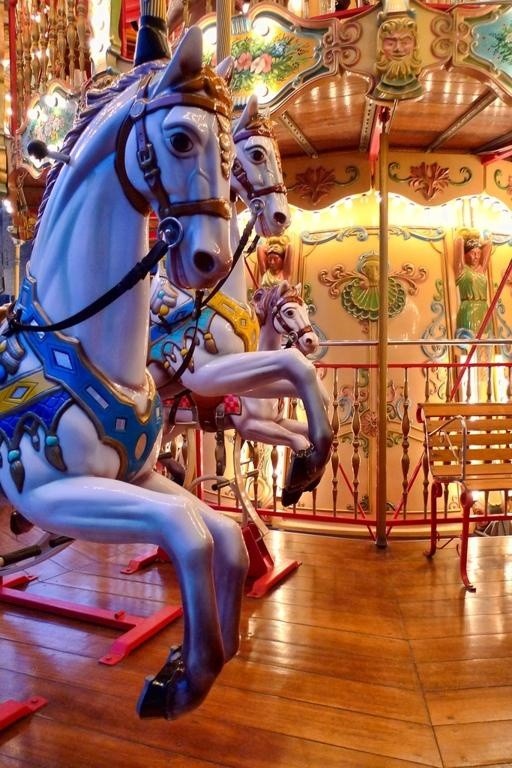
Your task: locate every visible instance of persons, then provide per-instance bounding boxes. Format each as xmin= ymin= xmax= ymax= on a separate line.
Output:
xmin=453 ymin=224 xmax=496 ymax=339
xmin=372 ymin=15 xmax=422 ymax=82
xmin=255 ymin=232 xmax=293 ymax=287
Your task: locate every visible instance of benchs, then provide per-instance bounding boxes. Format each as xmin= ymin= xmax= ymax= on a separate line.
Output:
xmin=416 ymin=403 xmax=512 ymax=593
